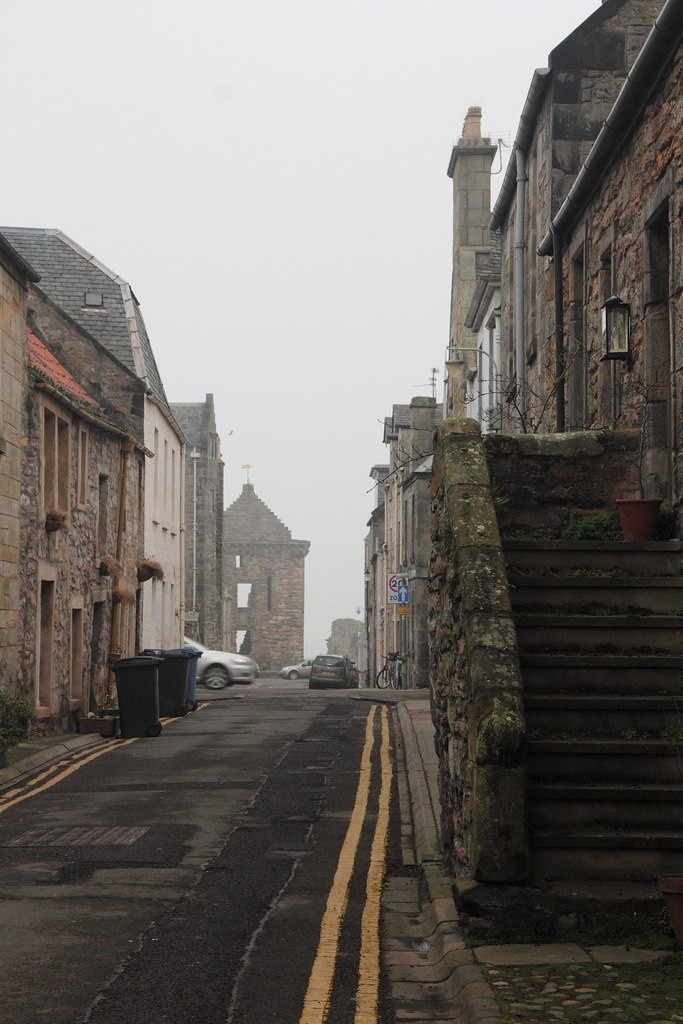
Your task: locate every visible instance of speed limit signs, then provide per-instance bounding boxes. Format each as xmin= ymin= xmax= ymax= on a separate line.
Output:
xmin=386 ymin=572 xmax=410 ymax=604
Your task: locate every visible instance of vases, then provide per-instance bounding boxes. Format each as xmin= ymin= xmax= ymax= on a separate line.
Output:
xmin=79 ymin=714 xmax=119 ymax=736
xmin=100 ymin=708 xmax=118 ymax=716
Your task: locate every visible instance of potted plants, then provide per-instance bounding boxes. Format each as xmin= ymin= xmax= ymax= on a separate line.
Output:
xmin=602 ymin=363 xmax=667 ymax=543
xmin=99 ymin=555 xmax=123 ymax=577
xmin=137 ymin=559 xmax=166 ymax=583
xmin=112 ymin=588 xmax=137 ymax=606
xmin=45 ymin=510 xmax=67 ymax=533
xmin=109 ymin=643 xmax=124 ymax=664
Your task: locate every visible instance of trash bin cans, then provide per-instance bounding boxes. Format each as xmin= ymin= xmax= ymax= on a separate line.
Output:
xmin=111 ymin=656 xmax=164 ymax=739
xmin=145 ymin=649 xmax=204 ymax=719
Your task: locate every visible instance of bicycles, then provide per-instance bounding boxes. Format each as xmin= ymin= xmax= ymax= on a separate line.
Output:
xmin=376 ymin=654 xmax=404 ymax=690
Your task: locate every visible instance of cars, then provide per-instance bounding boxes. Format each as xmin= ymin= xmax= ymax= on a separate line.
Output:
xmin=184 ymin=634 xmax=260 ymax=690
xmin=279 ymin=659 xmax=332 ymax=681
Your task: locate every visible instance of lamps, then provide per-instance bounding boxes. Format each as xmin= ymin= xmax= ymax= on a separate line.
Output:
xmin=364 ymin=570 xmax=372 ymax=582
xmin=597 ymin=293 xmax=634 ymax=362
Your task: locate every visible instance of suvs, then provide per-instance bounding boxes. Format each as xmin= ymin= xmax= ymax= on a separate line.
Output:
xmin=308 ymin=654 xmax=360 ymax=689
